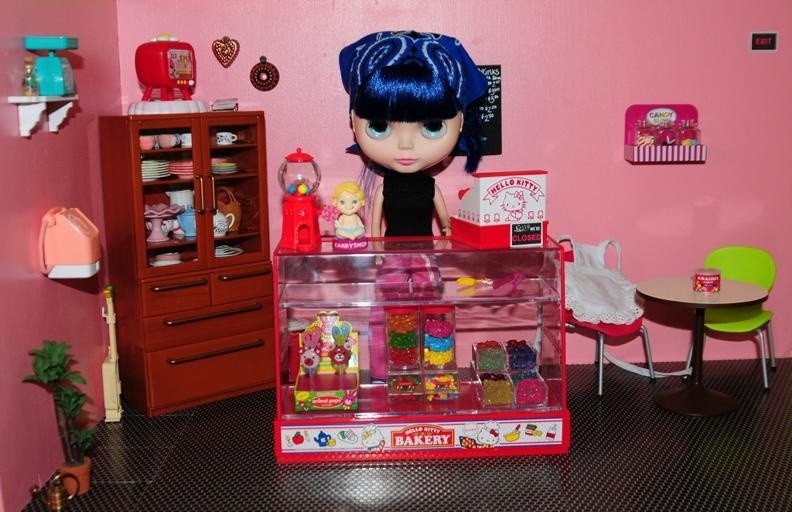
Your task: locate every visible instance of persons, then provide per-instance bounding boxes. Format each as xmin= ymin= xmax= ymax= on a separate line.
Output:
xmin=333 ymin=32 xmax=489 ymax=296
xmin=332 ymin=183 xmax=367 ymax=240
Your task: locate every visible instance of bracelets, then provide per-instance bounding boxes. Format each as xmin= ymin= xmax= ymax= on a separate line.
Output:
xmin=443 ymin=226 xmax=451 ymax=229
